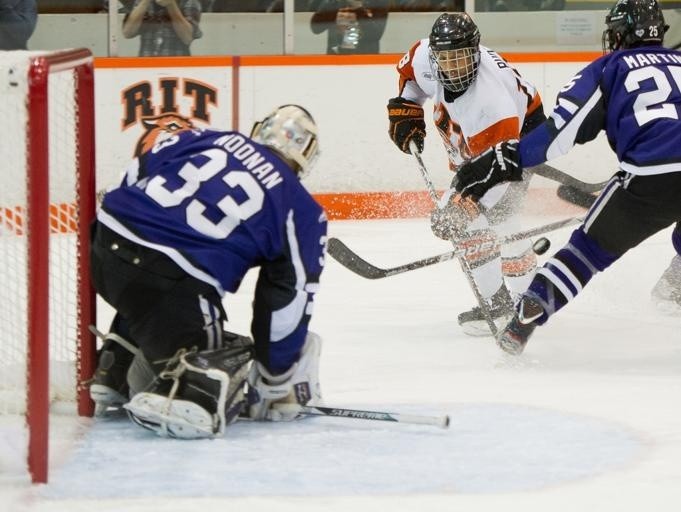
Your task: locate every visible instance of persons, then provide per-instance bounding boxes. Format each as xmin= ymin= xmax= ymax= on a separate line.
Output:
xmin=0 ymin=0 xmax=38 ymax=51
xmin=121 ymin=0 xmax=202 ymax=58
xmin=387 ymin=10 xmax=549 ymax=324
xmin=310 ymin=0 xmax=389 ymax=54
xmin=89 ymin=104 xmax=330 ymax=441
xmin=447 ymin=0 xmax=681 ymax=354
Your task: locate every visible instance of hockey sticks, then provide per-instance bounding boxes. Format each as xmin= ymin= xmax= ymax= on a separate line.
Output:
xmin=556 ymin=184 xmax=596 ymax=210
xmin=532 ymin=163 xmax=614 ymax=194
xmin=327 ymin=214 xmax=585 ymax=280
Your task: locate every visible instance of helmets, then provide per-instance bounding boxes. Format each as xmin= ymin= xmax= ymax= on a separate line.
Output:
xmin=602 ymin=0 xmax=666 ymax=57
xmin=250 ymin=102 xmax=321 ymax=176
xmin=428 ymin=12 xmax=482 ymax=54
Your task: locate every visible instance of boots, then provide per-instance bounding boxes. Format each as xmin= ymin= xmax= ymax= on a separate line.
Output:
xmin=90 ymin=326 xmax=139 ymax=405
xmin=650 ymin=256 xmax=681 ymax=306
xmin=128 ymin=346 xmax=228 ymax=440
xmin=456 ymin=283 xmax=546 ymax=354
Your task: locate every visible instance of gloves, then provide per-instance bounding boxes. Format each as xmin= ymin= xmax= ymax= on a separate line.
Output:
xmin=385 ymin=98 xmax=427 ymax=160
xmin=247 ymin=328 xmax=323 ymax=423
xmin=430 ymin=191 xmax=488 ymax=240
xmin=451 ymin=136 xmax=526 ymax=202
